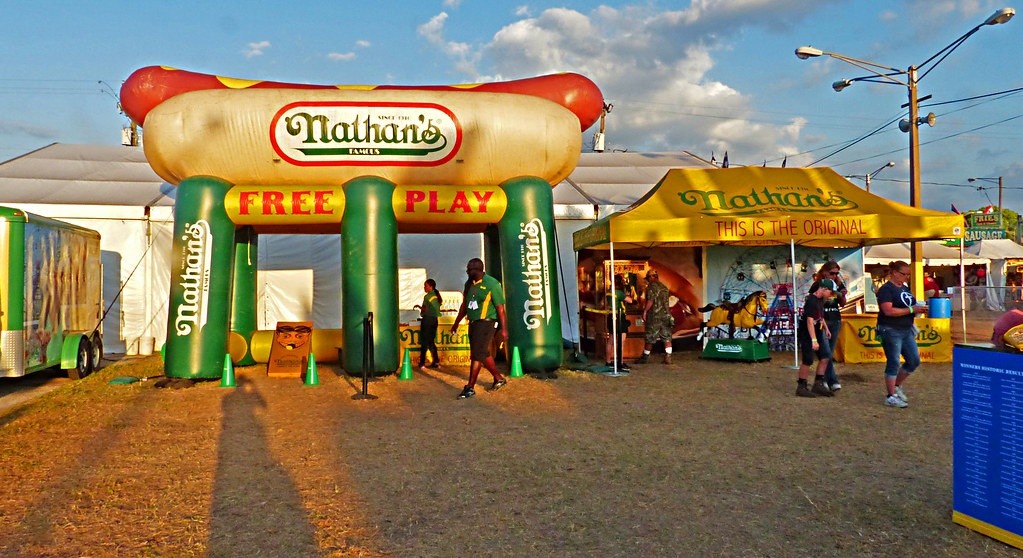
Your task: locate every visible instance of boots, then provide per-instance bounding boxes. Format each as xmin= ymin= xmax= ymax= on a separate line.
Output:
xmin=635 ymin=354 xmax=649 ymax=364
xmin=661 ymin=353 xmax=672 ymax=364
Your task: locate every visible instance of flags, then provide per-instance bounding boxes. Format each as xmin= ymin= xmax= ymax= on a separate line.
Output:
xmin=951 ymin=203 xmax=967 ymax=227
xmin=984 ymin=206 xmax=993 ymax=213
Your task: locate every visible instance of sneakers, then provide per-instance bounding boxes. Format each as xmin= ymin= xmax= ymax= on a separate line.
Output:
xmin=820 ymin=381 xmax=841 ymax=391
xmin=487 ymin=379 xmax=506 ymax=392
xmin=895 ymin=386 xmax=908 ymax=402
xmin=457 ymin=386 xmax=475 ymax=399
xmin=795 ymin=385 xmax=817 ymax=398
xmin=812 ymin=381 xmax=835 ymax=397
xmin=885 ymin=395 xmax=908 ymax=408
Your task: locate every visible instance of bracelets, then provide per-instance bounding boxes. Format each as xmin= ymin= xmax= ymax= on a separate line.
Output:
xmin=812 ymin=339 xmax=817 ymax=342
xmin=908 ymin=306 xmax=914 ymax=314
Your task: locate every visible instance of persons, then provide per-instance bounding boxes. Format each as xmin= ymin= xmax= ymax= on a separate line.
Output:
xmin=634 ymin=269 xmax=672 ymax=365
xmin=451 ymin=258 xmax=507 ymax=400
xmin=923 ymin=271 xmax=939 ymax=305
xmin=876 ymin=261 xmax=929 ymax=408
xmin=816 ymin=260 xmax=847 ymax=391
xmin=991 ymin=301 xmax=1023 ymax=347
xmin=796 ymin=278 xmax=837 ymax=397
xmin=606 ymin=275 xmax=632 ymax=371
xmin=415 ymin=279 xmax=443 ymax=369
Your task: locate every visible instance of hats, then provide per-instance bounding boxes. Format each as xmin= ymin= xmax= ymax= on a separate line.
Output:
xmin=643 ymin=269 xmax=658 ymax=279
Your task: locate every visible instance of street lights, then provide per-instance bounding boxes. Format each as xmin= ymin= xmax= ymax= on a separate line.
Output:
xmin=967 ymin=176 xmax=1002 ymax=226
xmin=845 ymin=161 xmax=895 ymax=192
xmin=795 ymin=9 xmax=1014 ymax=315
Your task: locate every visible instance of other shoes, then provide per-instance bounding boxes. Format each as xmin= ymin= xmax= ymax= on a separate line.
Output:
xmin=429 ymin=363 xmax=438 ymax=368
xmin=413 ymin=363 xmax=426 ymax=369
xmin=606 ymin=361 xmax=631 ymax=370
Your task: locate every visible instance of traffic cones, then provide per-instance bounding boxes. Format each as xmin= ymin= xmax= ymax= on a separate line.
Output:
xmin=218 ymin=354 xmax=239 ymax=388
xmin=507 ymin=346 xmax=526 ymax=379
xmin=398 ymin=350 xmax=416 ymax=381
xmin=303 ymin=352 xmax=322 ymax=387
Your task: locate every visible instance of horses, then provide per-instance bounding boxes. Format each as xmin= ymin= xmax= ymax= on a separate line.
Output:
xmin=697 ymin=290 xmax=773 ymax=343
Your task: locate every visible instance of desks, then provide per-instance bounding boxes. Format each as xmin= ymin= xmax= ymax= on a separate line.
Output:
xmin=834 ymin=314 xmax=953 ymax=365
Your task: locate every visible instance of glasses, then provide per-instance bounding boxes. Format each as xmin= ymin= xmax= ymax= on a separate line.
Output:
xmin=828 ymin=271 xmax=840 ymax=275
xmin=897 ymin=270 xmax=911 ymax=277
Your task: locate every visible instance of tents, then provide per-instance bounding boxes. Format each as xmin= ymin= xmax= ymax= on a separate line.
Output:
xmin=572 ymin=167 xmax=966 ymax=374
xmin=864 ymin=239 xmax=1023 ymax=312
xmin=0 ymin=143 xmax=721 ymax=355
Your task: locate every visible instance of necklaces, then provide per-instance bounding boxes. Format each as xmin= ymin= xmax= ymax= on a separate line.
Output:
xmin=897 ymin=285 xmax=904 ymax=292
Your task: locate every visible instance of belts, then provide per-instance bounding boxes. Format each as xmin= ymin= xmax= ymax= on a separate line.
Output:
xmin=471 ymin=318 xmax=494 ymax=322
